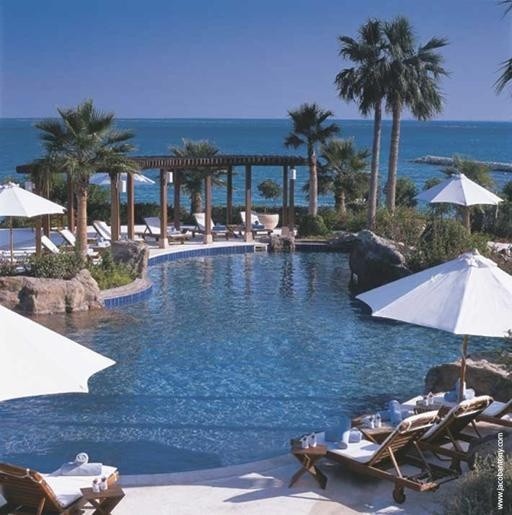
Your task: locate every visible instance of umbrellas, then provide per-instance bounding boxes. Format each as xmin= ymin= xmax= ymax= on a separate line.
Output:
xmin=1 ymin=303 xmax=115 ymax=404
xmin=0 ymin=180 xmax=68 ymax=261
xmin=87 ymin=171 xmax=157 ymax=187
xmin=413 ymin=170 xmax=504 ymax=223
xmin=355 ymin=250 xmax=512 ymax=405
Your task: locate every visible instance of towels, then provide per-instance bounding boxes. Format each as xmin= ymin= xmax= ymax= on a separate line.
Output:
xmin=75 ymin=452 xmax=88 ymax=463
xmin=61 ymin=462 xmax=102 ymax=476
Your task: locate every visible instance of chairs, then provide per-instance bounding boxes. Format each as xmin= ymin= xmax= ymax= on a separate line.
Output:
xmin=92 ymin=220 xmax=144 ymax=244
xmin=327 ymin=388 xmax=512 ymax=503
xmin=60 ymin=230 xmax=110 ymax=250
xmin=41 ymin=235 xmax=99 ymax=259
xmin=0 ymin=462 xmax=119 ymax=515
xmin=237 ymin=211 xmax=273 ymax=239
xmin=134 ymin=217 xmax=189 ymax=244
xmin=180 ymin=213 xmax=230 ymax=241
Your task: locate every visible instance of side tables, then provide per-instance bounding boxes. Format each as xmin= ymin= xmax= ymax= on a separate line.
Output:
xmin=288 ymin=439 xmax=327 ymax=489
xmin=80 ymin=484 xmax=125 ymax=515
